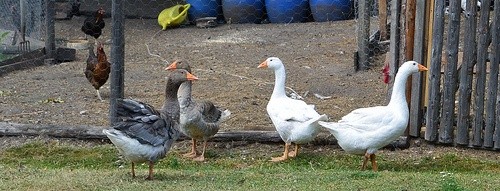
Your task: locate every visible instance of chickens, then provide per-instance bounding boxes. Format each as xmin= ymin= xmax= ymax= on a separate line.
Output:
xmin=79 ymin=8 xmax=106 ymax=46
xmin=84 ymin=44 xmax=110 ymax=102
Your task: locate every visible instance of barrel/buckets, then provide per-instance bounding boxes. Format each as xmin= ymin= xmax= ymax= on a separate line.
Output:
xmin=187 ymin=0 xmax=220 ymax=25
xmin=265 ymin=0 xmax=310 ymax=23
xmin=221 ymin=0 xmax=267 ymax=23
xmin=308 ymin=0 xmax=355 ymax=22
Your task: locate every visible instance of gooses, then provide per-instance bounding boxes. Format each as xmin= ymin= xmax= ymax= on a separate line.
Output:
xmin=316 ymin=60 xmax=429 ymax=172
xmin=256 ymin=56 xmax=328 ymax=162
xmin=102 ymin=59 xmax=232 ymax=181
